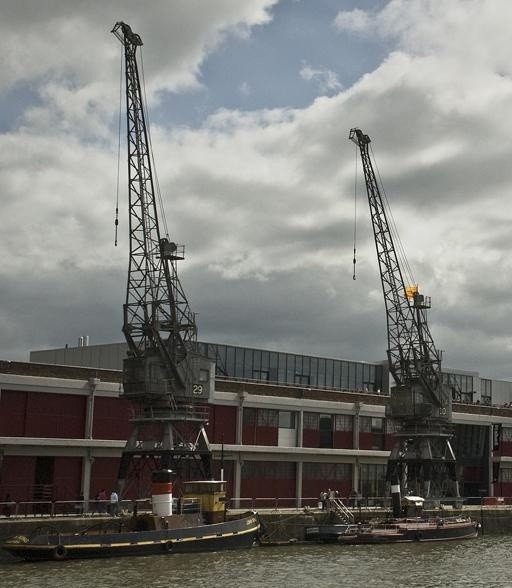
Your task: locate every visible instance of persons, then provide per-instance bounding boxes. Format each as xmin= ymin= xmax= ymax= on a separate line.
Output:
xmin=97 ymin=489 xmax=109 ymax=513
xmin=318 ymin=486 xmax=361 ymax=511
xmin=109 ymin=488 xmax=120 ymax=517
xmin=76 ymin=491 xmax=85 ymax=514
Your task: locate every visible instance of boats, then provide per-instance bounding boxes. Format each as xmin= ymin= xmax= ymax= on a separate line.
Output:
xmin=303 ymin=511 xmax=481 ymax=545
xmin=0 ymin=510 xmax=260 ymax=563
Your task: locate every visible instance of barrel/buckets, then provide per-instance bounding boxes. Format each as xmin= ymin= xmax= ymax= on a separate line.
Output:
xmin=151 ymin=494 xmax=173 ymax=517
